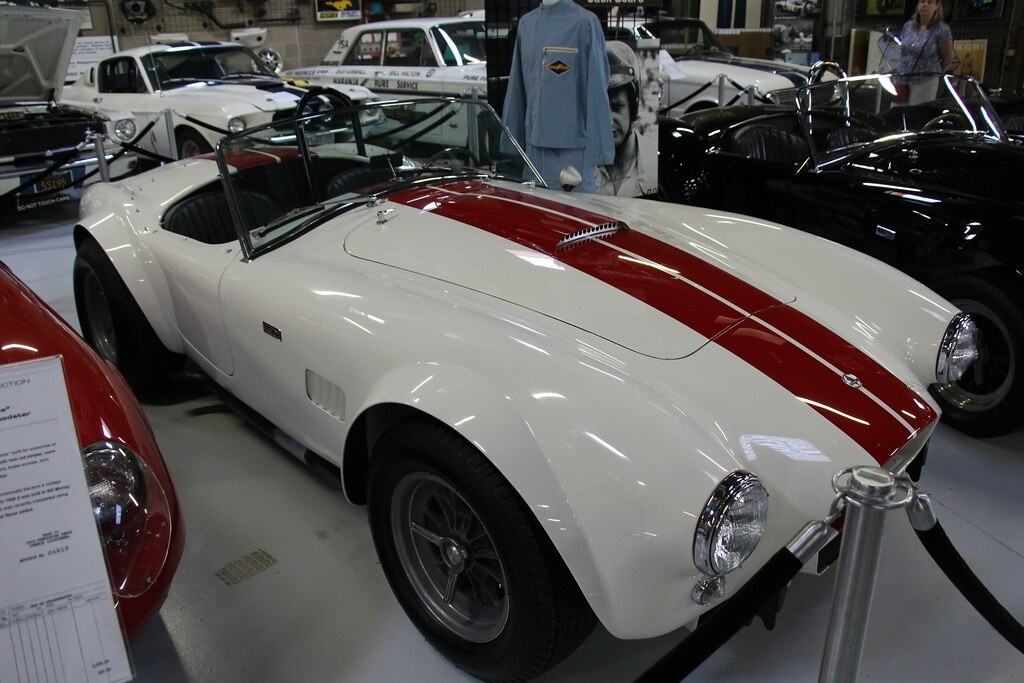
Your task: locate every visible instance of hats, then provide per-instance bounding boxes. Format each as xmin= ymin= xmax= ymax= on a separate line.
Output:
xmin=606 ymin=40 xmax=638 ymax=89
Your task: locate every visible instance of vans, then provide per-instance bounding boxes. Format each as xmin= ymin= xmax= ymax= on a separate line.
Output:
xmin=0 ymin=4 xmax=138 ymax=226
xmin=442 ymin=8 xmax=840 ymax=127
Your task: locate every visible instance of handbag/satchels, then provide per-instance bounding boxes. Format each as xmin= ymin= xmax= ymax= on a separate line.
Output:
xmin=891 ymin=84 xmax=909 ymax=102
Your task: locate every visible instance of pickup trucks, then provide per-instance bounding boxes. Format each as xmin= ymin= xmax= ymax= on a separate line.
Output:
xmin=59 ymin=40 xmax=385 ymax=162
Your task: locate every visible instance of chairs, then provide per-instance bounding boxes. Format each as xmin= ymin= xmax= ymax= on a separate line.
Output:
xmin=163 ymin=191 xmax=274 ymax=245
xmin=826 ymin=126 xmax=880 ymax=150
xmin=323 ymin=168 xmax=400 ymax=201
xmin=732 ymin=126 xmax=794 ymax=161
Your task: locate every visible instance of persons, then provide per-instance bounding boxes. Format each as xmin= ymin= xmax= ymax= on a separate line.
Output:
xmin=591 ymin=39 xmax=659 ymax=196
xmin=891 ymin=0 xmax=952 ymax=109
xmin=499 ymin=0 xmax=616 ymax=194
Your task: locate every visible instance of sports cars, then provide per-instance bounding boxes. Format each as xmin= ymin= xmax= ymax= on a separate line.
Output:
xmin=72 ymin=86 xmax=980 ymax=682
xmin=655 ymin=56 xmax=1024 ymax=440
xmin=1 ymin=260 xmax=187 ymax=653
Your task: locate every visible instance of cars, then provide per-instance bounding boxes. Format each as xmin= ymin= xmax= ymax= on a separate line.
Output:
xmin=281 ymin=18 xmax=493 ymax=159
